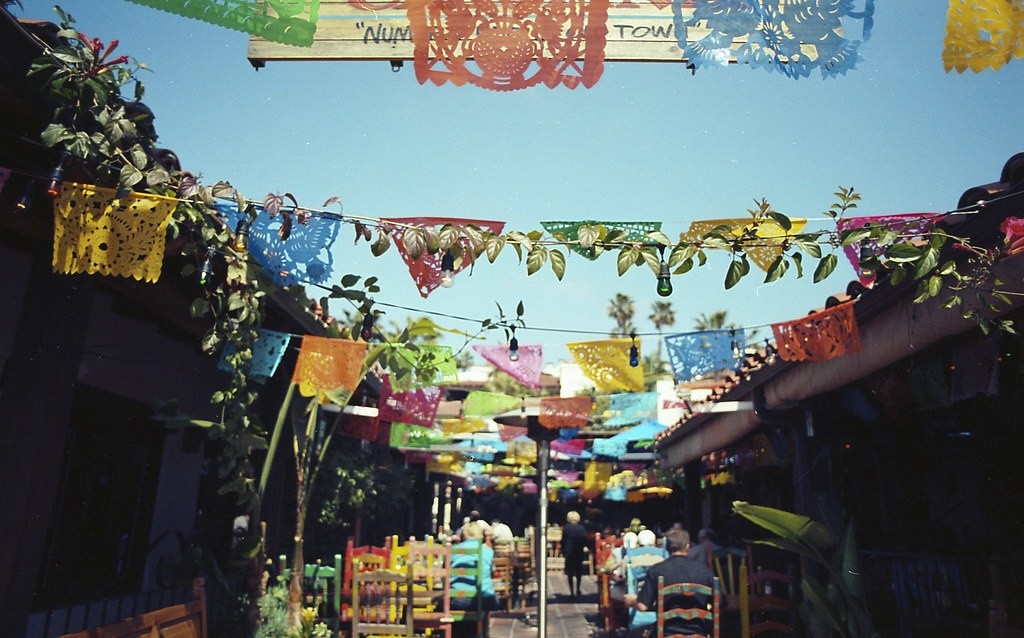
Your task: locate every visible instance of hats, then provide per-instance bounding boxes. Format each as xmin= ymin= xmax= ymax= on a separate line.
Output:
xmin=623 ymin=532 xmax=638 ymax=549
xmin=638 ymin=530 xmax=656 ymax=547
xmin=699 ymin=527 xmax=715 ymax=537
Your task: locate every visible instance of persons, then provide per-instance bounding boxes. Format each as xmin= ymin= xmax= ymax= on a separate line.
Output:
xmin=439 ymin=523 xmax=499 ymax=638
xmin=623 ymin=531 xmax=728 ymax=638
xmin=562 ymin=511 xmax=588 ymax=599
xmin=437 ymin=511 xmax=513 ymax=546
xmin=603 ymin=518 xmax=727 ymax=578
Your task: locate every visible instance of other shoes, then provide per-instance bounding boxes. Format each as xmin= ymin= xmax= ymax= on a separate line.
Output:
xmin=568 ymin=594 xmax=574 ymax=599
xmin=577 ymin=590 xmax=582 ymax=599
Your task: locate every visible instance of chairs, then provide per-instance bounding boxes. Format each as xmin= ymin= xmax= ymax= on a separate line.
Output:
xmin=277 ymin=535 xmax=531 ymax=638
xmin=593 ymin=530 xmax=805 ymax=638
